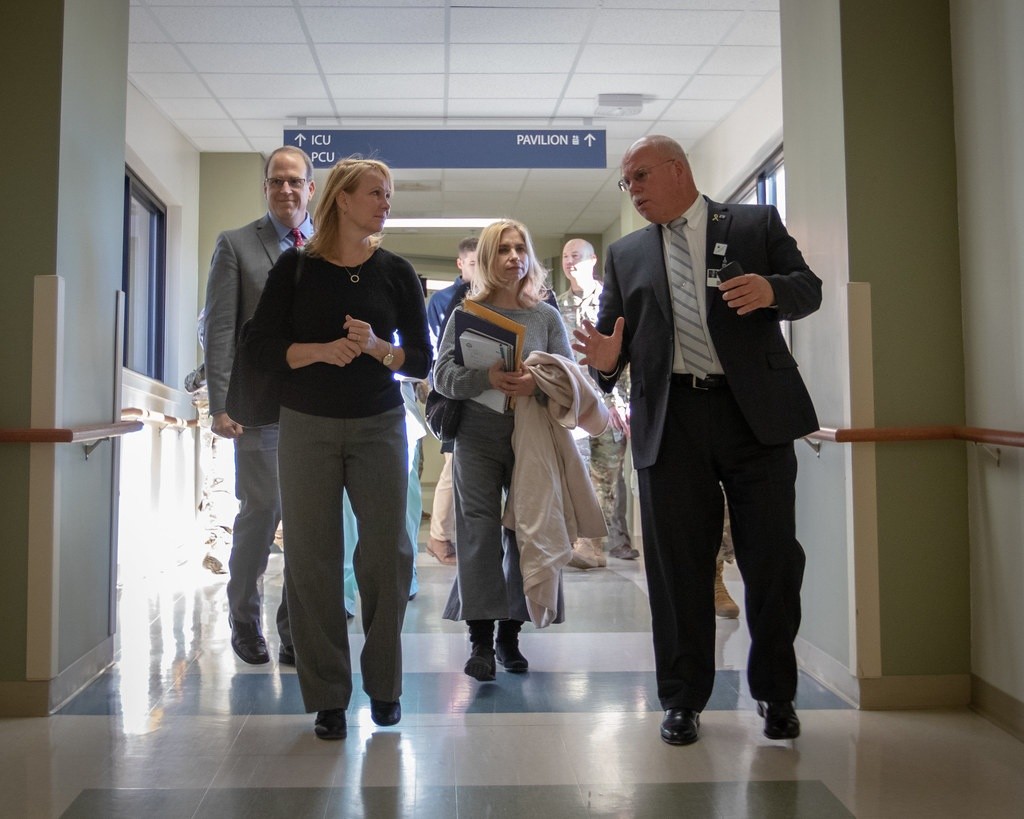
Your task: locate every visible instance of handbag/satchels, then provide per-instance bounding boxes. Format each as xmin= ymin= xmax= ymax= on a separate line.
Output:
xmin=226 ymin=251 xmax=304 ymax=427
xmin=426 ymin=390 xmax=461 ymax=453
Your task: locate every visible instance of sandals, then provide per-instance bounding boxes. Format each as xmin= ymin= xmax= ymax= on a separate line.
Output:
xmin=425 ymin=537 xmax=456 ymax=565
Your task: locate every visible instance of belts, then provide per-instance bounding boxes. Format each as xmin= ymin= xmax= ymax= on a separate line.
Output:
xmin=669 ymin=373 xmax=729 ymax=390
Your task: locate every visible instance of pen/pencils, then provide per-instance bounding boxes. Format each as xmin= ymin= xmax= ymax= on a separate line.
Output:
xmin=499 ymin=343 xmax=507 ymax=371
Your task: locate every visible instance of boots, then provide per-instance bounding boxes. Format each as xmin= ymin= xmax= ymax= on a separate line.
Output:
xmin=714 ymin=560 xmax=739 ymax=618
xmin=496 ymin=620 xmax=528 ymax=672
xmin=465 ymin=619 xmax=496 ymax=681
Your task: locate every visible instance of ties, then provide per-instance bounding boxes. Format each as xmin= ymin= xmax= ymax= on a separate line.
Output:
xmin=667 ymin=217 xmax=713 ymax=380
xmin=292 ymin=227 xmax=303 ymax=247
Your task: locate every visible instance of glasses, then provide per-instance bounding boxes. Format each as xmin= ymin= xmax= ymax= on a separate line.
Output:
xmin=619 ymin=159 xmax=675 ymax=192
xmin=268 ymin=177 xmax=305 ymax=187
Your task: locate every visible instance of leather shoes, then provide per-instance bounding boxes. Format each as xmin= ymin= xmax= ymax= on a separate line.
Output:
xmin=279 ymin=653 xmax=296 ymax=665
xmin=231 ymin=615 xmax=269 ymax=665
xmin=758 ymin=701 xmax=800 ymax=740
xmin=661 ymin=708 xmax=700 ymax=746
xmin=371 ymin=699 xmax=401 ymax=726
xmin=315 ymin=709 xmax=347 ymax=740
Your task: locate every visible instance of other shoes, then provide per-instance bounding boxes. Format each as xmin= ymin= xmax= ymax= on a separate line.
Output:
xmin=569 ymin=538 xmax=606 ymax=571
xmin=610 ymin=544 xmax=640 ymax=559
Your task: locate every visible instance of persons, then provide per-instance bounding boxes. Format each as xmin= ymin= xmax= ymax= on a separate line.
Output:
xmin=554 ymin=237 xmax=640 ymax=569
xmin=428 ymin=238 xmax=477 ymax=564
xmin=433 ymin=221 xmax=575 ymax=682
xmin=203 ymin=146 xmax=317 ymax=666
xmin=244 ymin=158 xmax=413 ymax=741
xmin=572 ymin=134 xmax=823 ymax=749
xmin=343 ymin=272 xmax=424 ymax=616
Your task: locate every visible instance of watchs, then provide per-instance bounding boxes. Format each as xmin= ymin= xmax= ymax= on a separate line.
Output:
xmin=384 ymin=342 xmax=393 ymax=367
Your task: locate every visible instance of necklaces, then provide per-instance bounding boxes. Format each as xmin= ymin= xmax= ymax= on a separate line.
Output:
xmin=335 ymin=250 xmax=369 ymax=282
